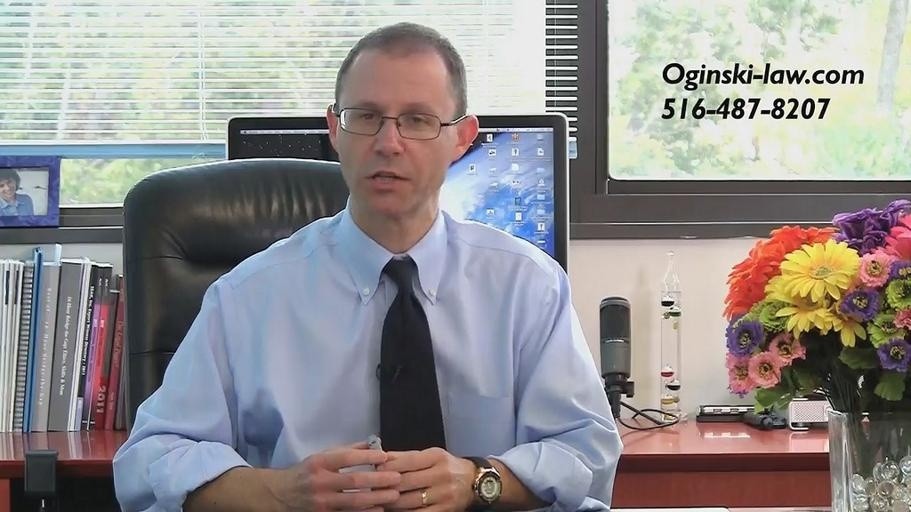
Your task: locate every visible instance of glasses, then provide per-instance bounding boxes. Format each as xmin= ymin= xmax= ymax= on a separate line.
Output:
xmin=333 ymin=104 xmax=467 ymax=141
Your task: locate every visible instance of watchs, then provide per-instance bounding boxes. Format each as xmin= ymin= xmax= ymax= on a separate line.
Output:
xmin=458 ymin=456 xmax=502 ymax=511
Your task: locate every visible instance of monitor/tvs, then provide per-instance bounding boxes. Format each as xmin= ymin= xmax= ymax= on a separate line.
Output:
xmin=226 ymin=112 xmax=569 ymax=273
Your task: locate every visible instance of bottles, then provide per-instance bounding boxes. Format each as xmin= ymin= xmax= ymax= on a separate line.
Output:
xmin=660 ymin=254 xmax=682 ymax=420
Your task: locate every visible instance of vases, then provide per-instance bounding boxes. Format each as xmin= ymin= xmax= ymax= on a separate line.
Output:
xmin=824 ymin=408 xmax=911 ymax=510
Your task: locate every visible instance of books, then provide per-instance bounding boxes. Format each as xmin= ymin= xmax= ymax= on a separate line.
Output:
xmin=0 ymin=244 xmax=128 ymax=435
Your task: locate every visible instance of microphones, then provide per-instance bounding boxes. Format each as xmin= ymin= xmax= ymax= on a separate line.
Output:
xmin=598 ymin=295 xmax=633 ymax=420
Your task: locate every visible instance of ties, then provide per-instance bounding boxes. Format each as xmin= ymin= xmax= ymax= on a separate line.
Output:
xmin=380 ymin=258 xmax=446 ymax=451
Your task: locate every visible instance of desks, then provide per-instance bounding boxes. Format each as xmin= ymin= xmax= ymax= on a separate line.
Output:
xmin=2 ymin=413 xmax=828 ymax=508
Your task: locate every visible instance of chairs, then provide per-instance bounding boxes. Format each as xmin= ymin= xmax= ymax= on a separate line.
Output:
xmin=119 ymin=154 xmax=350 ymax=443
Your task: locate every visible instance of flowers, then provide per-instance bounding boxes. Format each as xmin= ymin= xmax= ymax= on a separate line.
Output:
xmin=723 ymin=196 xmax=911 ymax=509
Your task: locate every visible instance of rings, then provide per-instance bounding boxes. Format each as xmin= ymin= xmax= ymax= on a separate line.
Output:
xmin=419 ymin=489 xmax=430 ymax=505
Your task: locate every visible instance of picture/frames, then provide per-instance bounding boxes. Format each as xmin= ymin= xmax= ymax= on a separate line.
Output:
xmin=1 ymin=154 xmax=64 ymax=231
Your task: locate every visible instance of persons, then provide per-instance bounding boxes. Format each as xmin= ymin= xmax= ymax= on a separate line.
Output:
xmin=113 ymin=18 xmax=622 ymax=512
xmin=1 ymin=168 xmax=35 ymax=218
